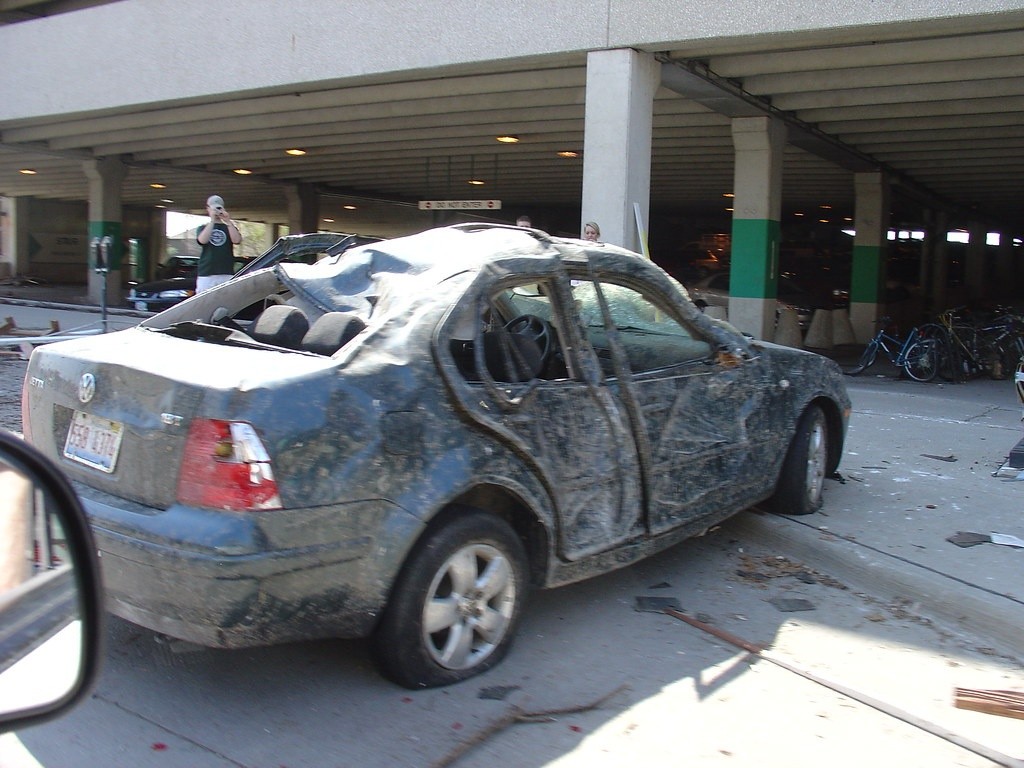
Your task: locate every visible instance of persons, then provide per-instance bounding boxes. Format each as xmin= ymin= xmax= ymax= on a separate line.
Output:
xmin=584 ymin=221 xmax=601 ymax=242
xmin=517 ymin=214 xmax=532 ymax=229
xmin=197 ymin=195 xmax=242 ymax=296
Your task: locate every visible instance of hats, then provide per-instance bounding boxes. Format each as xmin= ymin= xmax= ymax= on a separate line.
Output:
xmin=207 ymin=195 xmax=224 ymax=209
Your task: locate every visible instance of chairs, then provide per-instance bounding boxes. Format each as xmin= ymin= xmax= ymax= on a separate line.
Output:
xmin=247 ymin=304 xmax=365 ymax=356
xmin=469 ymin=332 xmax=543 ymax=383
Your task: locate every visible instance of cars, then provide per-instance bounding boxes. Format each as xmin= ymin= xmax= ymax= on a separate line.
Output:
xmin=683 ymin=268 xmax=823 ymax=335
xmin=156 ymin=252 xmax=200 ymax=279
xmin=31 ymin=221 xmax=850 ymax=687
xmin=127 ymin=257 xmax=262 ymax=323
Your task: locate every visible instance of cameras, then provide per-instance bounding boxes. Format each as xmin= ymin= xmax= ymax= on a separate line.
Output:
xmin=216 ymin=207 xmax=223 ymax=214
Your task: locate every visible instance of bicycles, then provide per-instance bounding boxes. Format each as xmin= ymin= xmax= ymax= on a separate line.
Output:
xmin=842 ymin=304 xmax=1024 ymax=386
